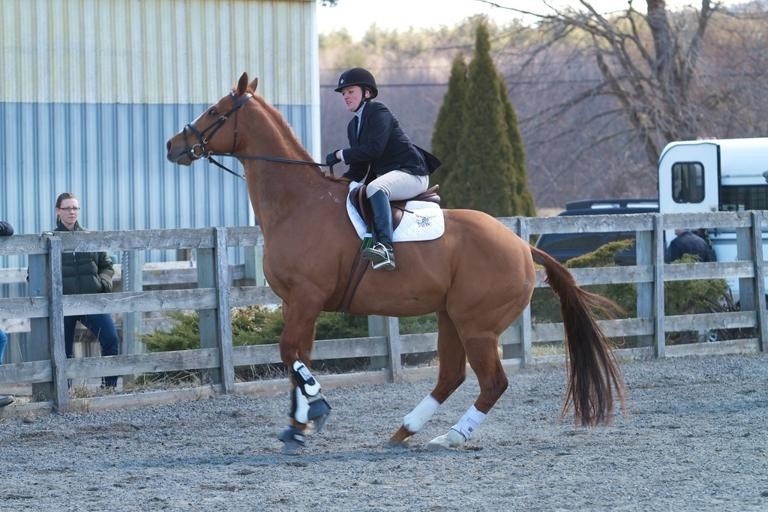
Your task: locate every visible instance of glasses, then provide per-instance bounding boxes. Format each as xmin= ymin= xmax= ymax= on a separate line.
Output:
xmin=59 ymin=208 xmax=80 ymax=211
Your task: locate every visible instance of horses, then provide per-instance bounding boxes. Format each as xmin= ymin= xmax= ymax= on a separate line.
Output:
xmin=164 ymin=71 xmax=627 ymax=456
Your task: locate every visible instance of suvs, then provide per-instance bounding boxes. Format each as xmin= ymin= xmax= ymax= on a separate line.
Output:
xmin=529 ymin=197 xmax=724 ymax=349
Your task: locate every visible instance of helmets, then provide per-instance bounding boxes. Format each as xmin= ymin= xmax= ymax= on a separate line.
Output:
xmin=335 ymin=68 xmax=377 ymax=98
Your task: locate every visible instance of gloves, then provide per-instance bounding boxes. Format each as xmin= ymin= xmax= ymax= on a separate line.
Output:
xmin=325 ymin=150 xmax=340 ymax=166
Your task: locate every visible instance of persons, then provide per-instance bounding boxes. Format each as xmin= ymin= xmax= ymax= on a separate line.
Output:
xmin=0 ymin=220 xmax=14 ymax=409
xmin=666 ymin=229 xmax=713 ymax=262
xmin=26 ymin=193 xmax=120 ymax=389
xmin=326 ymin=67 xmax=441 ymax=271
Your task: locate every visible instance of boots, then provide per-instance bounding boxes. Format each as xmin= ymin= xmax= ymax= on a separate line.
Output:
xmin=361 ymin=191 xmax=396 ymax=271
